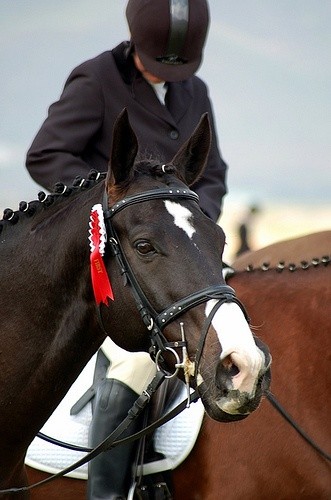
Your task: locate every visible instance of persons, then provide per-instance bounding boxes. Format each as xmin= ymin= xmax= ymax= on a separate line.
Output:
xmin=24 ymin=0 xmax=234 ymax=500
xmin=237 ymin=204 xmax=267 ymax=263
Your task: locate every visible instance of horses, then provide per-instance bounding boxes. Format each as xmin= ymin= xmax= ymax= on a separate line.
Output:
xmin=0 ymin=107 xmax=331 ymax=500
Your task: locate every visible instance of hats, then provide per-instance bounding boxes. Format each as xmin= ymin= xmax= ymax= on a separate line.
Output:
xmin=125 ymin=0 xmax=210 ymax=83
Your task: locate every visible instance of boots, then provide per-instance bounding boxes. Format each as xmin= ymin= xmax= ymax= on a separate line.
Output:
xmin=87 ymin=379 xmax=147 ymax=500
